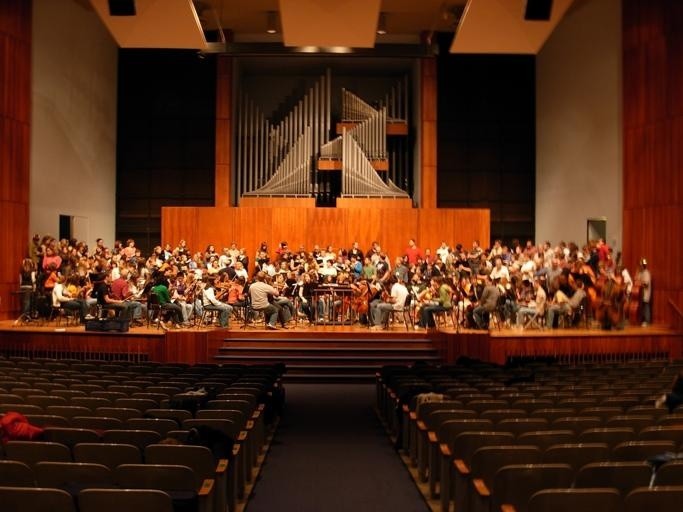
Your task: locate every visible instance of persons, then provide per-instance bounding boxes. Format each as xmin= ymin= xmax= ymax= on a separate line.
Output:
xmin=20 ymin=234 xmax=651 ymax=333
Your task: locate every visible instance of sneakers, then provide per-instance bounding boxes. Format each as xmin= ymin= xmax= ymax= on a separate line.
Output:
xmin=489 ymin=314 xmax=555 ymax=333
xmin=64 ymin=313 xmax=107 ymax=324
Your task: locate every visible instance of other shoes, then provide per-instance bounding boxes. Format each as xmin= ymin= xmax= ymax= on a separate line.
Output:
xmin=416 ymin=321 xmax=420 ymax=324
xmin=133 ymin=319 xmax=295 ymax=331
xmin=370 ymin=324 xmax=384 ymax=330
xmin=427 ymin=325 xmax=437 ymax=330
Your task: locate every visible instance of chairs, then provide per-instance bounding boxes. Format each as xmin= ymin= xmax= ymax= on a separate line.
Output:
xmin=22 ymin=284 xmax=586 ymax=332
xmin=374 ymin=360 xmax=683 ymax=511
xmin=0 ymin=355 xmax=287 ymax=512
